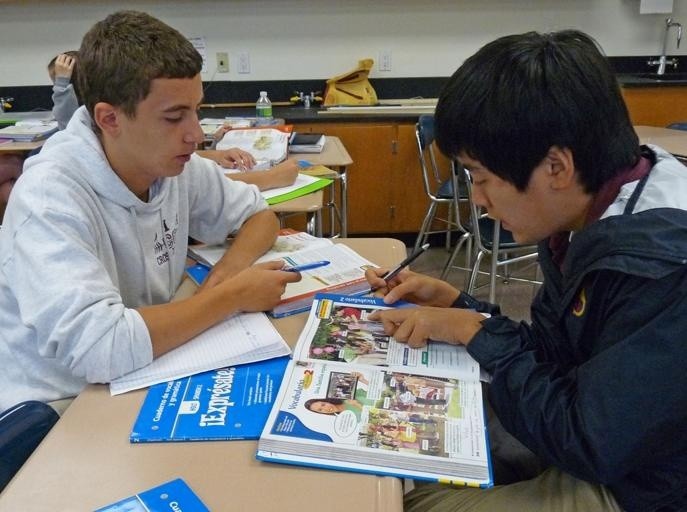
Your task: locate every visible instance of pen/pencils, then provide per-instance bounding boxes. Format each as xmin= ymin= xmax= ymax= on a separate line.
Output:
xmin=371 ymin=243 xmax=431 ymax=292
xmin=281 ymin=261 xmax=330 ymax=272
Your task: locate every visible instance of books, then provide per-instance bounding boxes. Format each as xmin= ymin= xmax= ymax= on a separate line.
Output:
xmin=91 ymin=475 xmax=209 ymax=511
xmin=199 ymin=115 xmax=339 ymax=207
xmin=253 ymin=292 xmax=494 ymax=491
xmin=0 ymin=120 xmax=59 ymax=147
xmin=106 ymin=310 xmax=294 ymax=399
xmin=186 ymin=226 xmax=385 ymax=320
xmin=185 ymin=263 xmax=211 ymax=287
xmin=129 ymin=347 xmax=292 ymax=445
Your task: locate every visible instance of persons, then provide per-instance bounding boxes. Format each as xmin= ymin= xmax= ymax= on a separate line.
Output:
xmin=0 ymin=51 xmax=84 ymax=208
xmin=303 ymin=398 xmax=361 ymax=427
xmin=362 ymin=26 xmax=683 ymax=510
xmin=0 ymin=8 xmax=301 ymax=421
xmin=368 ymin=370 xmax=451 ymax=453
xmin=190 ymin=148 xmax=298 ymax=192
xmin=311 ymin=306 xmax=390 ymax=366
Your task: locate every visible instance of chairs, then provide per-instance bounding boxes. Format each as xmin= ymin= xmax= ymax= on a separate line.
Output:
xmin=415 ymin=115 xmax=468 ymax=249
xmin=0 ymin=399 xmax=60 ymax=490
xmin=440 ymin=160 xmax=543 ymax=303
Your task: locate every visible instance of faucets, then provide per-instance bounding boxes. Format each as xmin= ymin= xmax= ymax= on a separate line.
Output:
xmin=661 ymin=23 xmax=682 ymax=56
xmin=304 ymin=96 xmax=310 ymax=108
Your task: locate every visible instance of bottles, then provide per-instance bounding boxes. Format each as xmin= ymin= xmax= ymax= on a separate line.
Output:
xmin=256 ymin=91 xmax=272 ymax=126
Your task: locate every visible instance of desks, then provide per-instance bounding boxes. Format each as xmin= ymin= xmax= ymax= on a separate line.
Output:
xmin=288 ymin=133 xmax=354 ymax=239
xmin=0 ymin=110 xmax=52 ymax=122
xmin=2 ymin=238 xmax=411 ymax=512
xmin=635 ymin=125 xmax=686 ymax=163
xmin=264 ymin=167 xmax=327 ymax=238
xmin=1 ymin=122 xmax=61 ymax=157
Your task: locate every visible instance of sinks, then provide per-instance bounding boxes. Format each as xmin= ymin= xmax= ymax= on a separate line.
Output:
xmin=633 ymin=74 xmax=687 ymax=81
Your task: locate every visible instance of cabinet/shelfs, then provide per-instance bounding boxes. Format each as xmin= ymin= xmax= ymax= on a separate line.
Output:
xmin=278 ymin=102 xmax=464 ymax=236
